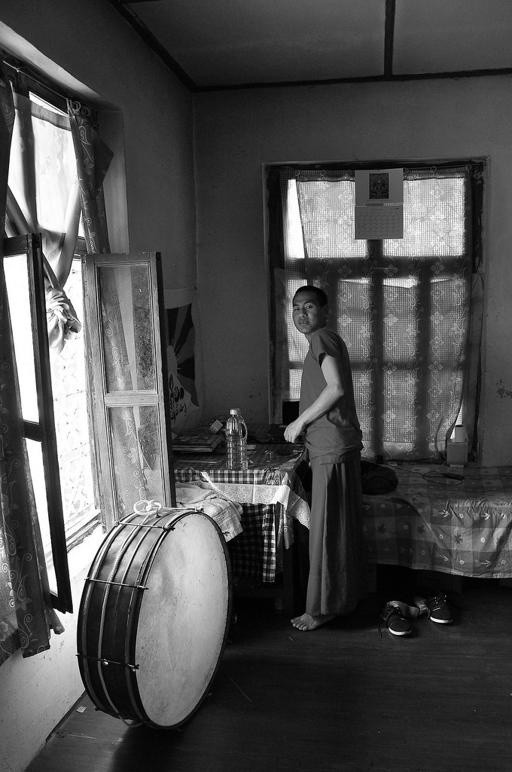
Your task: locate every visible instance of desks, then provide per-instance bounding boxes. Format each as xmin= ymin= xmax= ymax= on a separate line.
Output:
xmin=170 ymin=421 xmax=308 ymax=621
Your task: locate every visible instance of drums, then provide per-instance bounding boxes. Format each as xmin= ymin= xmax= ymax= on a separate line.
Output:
xmin=76 ymin=506 xmax=235 ymax=731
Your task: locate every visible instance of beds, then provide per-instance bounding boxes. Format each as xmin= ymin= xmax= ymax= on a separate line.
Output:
xmin=296 ymin=463 xmax=512 ymax=611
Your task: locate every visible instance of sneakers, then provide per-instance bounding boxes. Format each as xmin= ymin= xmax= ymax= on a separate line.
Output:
xmin=427 ymin=590 xmax=456 ymax=625
xmin=379 ymin=599 xmax=414 ymax=636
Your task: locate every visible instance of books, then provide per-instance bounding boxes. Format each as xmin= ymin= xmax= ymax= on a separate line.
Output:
xmin=170 ymin=430 xmax=222 ymax=453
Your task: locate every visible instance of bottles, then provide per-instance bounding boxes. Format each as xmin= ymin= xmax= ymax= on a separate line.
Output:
xmin=225 ymin=408 xmax=248 ymax=471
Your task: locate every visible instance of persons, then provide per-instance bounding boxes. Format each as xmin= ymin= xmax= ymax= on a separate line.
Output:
xmin=282 ymin=284 xmax=370 ymax=632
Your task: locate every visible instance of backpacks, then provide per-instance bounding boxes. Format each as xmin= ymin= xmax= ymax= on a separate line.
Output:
xmin=360 ymin=459 xmax=398 ymax=495
